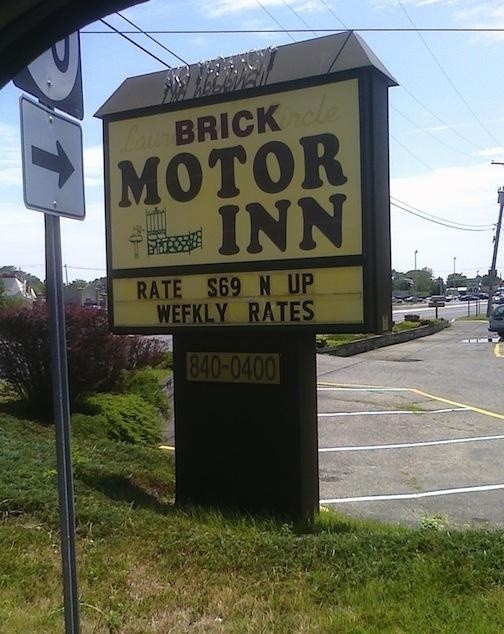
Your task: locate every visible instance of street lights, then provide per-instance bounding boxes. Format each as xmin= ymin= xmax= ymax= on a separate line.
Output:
xmin=477 ymin=270 xmax=479 ymax=277
xmin=414 ymin=250 xmax=418 ymax=271
xmin=454 ymin=257 xmax=456 ymax=291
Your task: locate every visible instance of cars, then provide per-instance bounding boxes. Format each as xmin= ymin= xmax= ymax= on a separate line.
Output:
xmin=392 ymin=295 xmax=451 ymax=307
xmin=460 ymin=293 xmax=489 ymax=301
xmin=488 ymin=287 xmax=504 ymax=339
